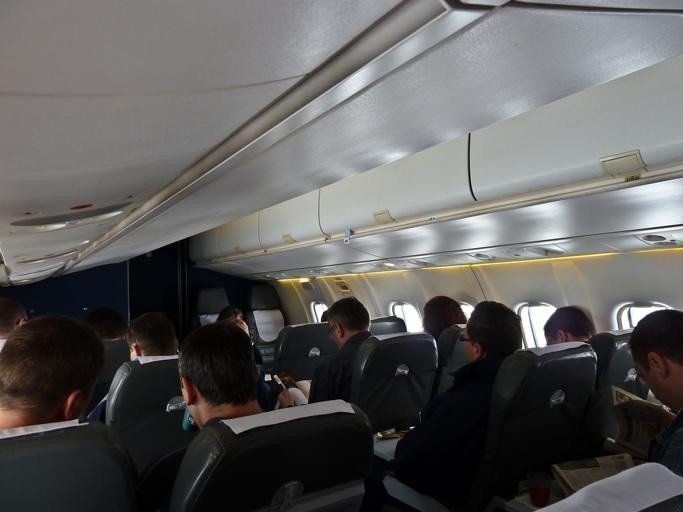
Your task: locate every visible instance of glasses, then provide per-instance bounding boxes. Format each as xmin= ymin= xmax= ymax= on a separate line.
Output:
xmin=327 ymin=324 xmax=334 ymax=337
xmin=455 ymin=331 xmax=471 ymax=342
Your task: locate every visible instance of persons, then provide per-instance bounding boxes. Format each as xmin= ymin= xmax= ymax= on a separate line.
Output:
xmin=269 ymin=299 xmax=380 ymax=416
xmin=409 ymin=297 xmax=467 ymax=371
xmin=214 ymin=308 xmax=256 ymax=347
xmin=167 ymin=319 xmax=274 ymax=468
xmin=1 ymin=296 xmax=109 ymax=437
xmin=543 ymin=305 xmax=596 ymax=346
xmin=113 ymin=313 xmax=180 ymax=370
xmin=626 ymin=307 xmax=683 ymax=512
xmin=362 ymin=301 xmax=526 ymax=512
